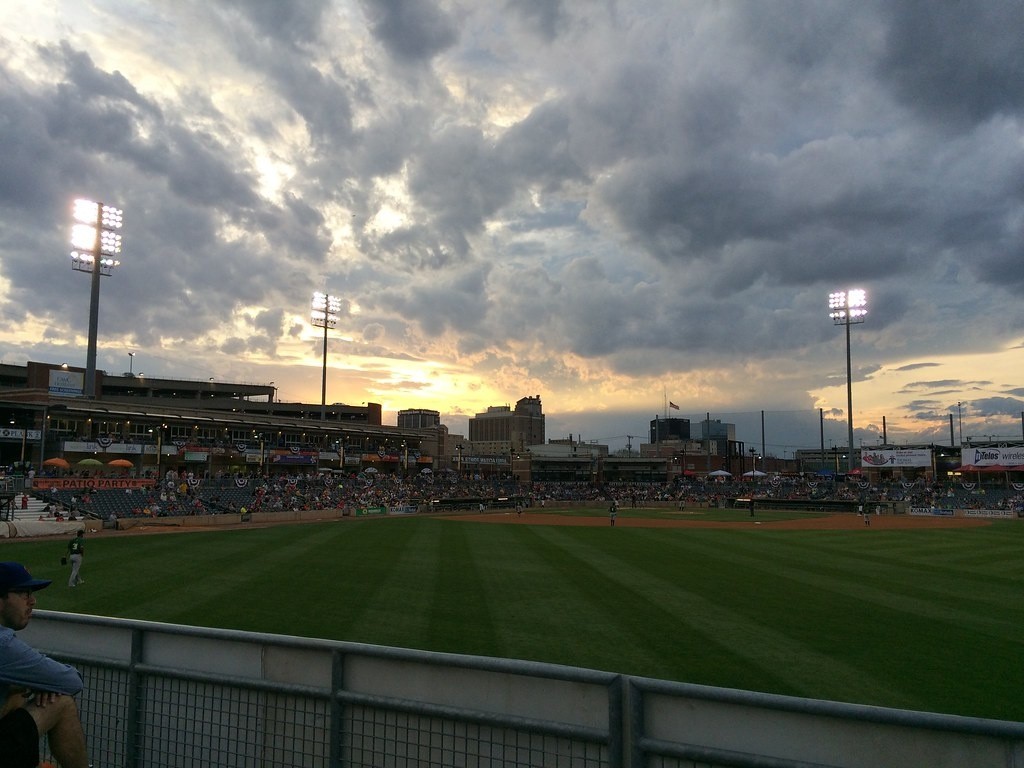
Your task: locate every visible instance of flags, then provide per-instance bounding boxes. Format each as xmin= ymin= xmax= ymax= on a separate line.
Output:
xmin=670 ymin=402 xmax=679 ymax=410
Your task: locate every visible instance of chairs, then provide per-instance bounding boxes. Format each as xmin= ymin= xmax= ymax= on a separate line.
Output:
xmin=24 ymin=479 xmax=525 ymax=519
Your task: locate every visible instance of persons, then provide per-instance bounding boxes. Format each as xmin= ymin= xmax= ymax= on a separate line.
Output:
xmin=759 ymin=472 xmax=1024 ymax=510
xmin=70 ymin=484 xmax=99 ymax=503
xmin=0 ymin=463 xmax=15 ymax=475
xmin=43 ymin=482 xmax=58 ymax=502
xmin=608 ymin=502 xmax=618 ymax=526
xmin=748 ymin=497 xmax=755 ymax=517
xmin=515 ymin=498 xmax=523 ymax=518
xmin=863 ymin=508 xmax=871 ymax=528
xmin=133 ymin=432 xmax=451 ymax=458
xmin=426 ymin=465 xmax=756 ymax=512
xmin=0 ymin=562 xmax=91 ymax=768
xmin=41 ymin=499 xmax=91 ymax=522
xmin=108 ymin=467 xmax=426 ymax=529
xmin=39 ymin=516 xmax=45 ymax=521
xmin=70 ymin=431 xmax=193 ymax=445
xmin=479 ymin=497 xmax=484 ymax=513
xmin=36 ymin=466 xmax=129 ymax=479
xmin=21 ymin=493 xmax=29 ymax=509
xmin=28 ymin=467 xmax=35 ymax=491
xmin=64 ymin=530 xmax=86 ymax=587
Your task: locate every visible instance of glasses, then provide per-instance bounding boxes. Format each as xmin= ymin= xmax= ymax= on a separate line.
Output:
xmin=8 ymin=588 xmax=34 ymax=598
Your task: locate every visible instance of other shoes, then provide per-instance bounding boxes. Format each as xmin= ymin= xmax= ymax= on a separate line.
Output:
xmin=68 ymin=584 xmax=76 ymax=587
xmin=77 ymin=580 xmax=85 ymax=584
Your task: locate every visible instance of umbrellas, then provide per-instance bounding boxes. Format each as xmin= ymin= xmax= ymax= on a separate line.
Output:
xmin=954 ymin=464 xmax=1024 ymax=472
xmin=709 ymin=470 xmax=732 ymax=475
xmin=743 ymin=470 xmax=766 ymax=476
xmin=44 ymin=457 xmax=134 ymax=467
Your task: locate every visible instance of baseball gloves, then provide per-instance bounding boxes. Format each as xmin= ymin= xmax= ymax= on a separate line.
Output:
xmin=61 ymin=556 xmax=67 ymax=566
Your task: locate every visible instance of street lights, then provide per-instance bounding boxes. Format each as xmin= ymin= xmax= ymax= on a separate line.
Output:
xmin=128 ymin=352 xmax=135 ymax=374
xmin=311 ymin=290 xmax=342 ymax=421
xmin=828 ymin=287 xmax=868 ymax=473
xmin=455 ymin=443 xmax=461 ymax=470
xmin=253 ymin=433 xmax=263 ymax=467
xmin=401 ymin=440 xmax=408 ymax=471
xmin=748 ymin=446 xmax=762 ymax=480
xmin=273 ymin=387 xmax=279 ymax=402
xmin=335 ymin=438 xmax=343 ymax=469
xmin=509 ymin=446 xmax=520 ymax=472
xmin=69 ymin=197 xmax=125 ymax=397
xmin=148 ymin=423 xmax=162 ymax=478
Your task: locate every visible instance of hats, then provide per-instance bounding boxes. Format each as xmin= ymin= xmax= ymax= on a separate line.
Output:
xmin=0 ymin=561 xmax=53 ymax=592
xmin=76 ymin=530 xmax=85 ymax=536
